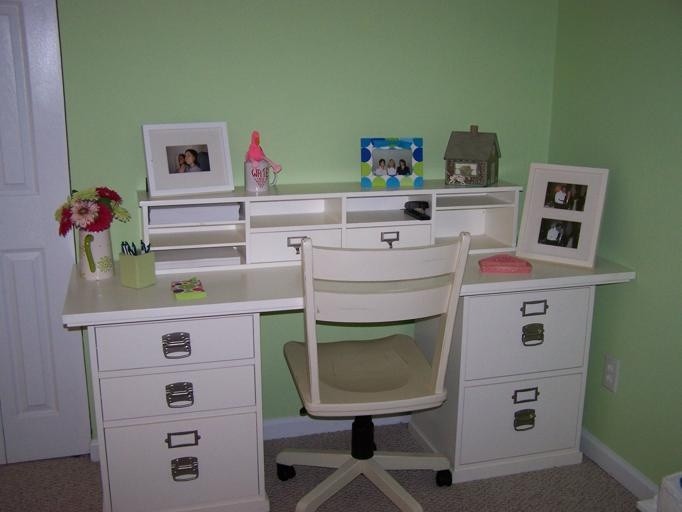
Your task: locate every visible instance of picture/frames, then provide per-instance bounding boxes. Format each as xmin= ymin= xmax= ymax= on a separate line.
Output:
xmin=139 ymin=120 xmax=235 ymax=197
xmin=515 ymin=162 xmax=611 ymax=268
xmin=360 ymin=136 xmax=424 ymax=190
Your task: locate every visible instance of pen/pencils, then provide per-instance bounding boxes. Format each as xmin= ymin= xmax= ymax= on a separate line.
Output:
xmin=121 ymin=240 xmax=151 ymax=256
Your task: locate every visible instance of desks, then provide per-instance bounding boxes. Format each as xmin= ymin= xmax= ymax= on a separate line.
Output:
xmin=61 ymin=250 xmax=636 ymax=512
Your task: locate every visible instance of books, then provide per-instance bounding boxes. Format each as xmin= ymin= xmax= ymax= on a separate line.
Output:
xmin=170 ymin=276 xmax=207 ymax=300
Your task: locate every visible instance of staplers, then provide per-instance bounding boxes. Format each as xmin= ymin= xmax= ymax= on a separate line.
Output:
xmin=402 ymin=200 xmax=431 ymax=221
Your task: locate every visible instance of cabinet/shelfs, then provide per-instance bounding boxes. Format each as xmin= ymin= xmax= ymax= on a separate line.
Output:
xmin=136 ymin=177 xmax=525 ymax=277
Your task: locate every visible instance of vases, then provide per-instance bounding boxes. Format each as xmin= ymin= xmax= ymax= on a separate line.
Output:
xmin=77 ymin=226 xmax=116 ymax=283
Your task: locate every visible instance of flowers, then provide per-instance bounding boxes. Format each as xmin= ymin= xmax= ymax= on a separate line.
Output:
xmin=54 ymin=186 xmax=130 ymax=238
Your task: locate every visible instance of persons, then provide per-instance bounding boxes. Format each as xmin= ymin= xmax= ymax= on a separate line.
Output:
xmin=387 ymin=159 xmax=396 ymax=175
xmin=177 ymin=154 xmax=186 ymax=172
xmin=564 ymin=191 xmax=573 ymax=209
xmin=375 ymin=158 xmax=387 ymax=175
xmin=554 ymin=186 xmax=566 ymax=209
xmin=397 ymin=159 xmax=409 ymax=175
xmin=556 ymin=229 xmax=568 ymax=247
xmin=184 ymin=149 xmax=201 ymax=172
xmin=546 ymin=223 xmax=559 ymax=245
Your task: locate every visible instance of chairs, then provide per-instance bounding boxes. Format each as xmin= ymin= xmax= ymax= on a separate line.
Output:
xmin=273 ymin=231 xmax=473 ymax=511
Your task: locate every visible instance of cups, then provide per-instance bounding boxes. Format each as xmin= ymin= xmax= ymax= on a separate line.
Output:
xmin=244 ymin=158 xmax=280 ymax=193
